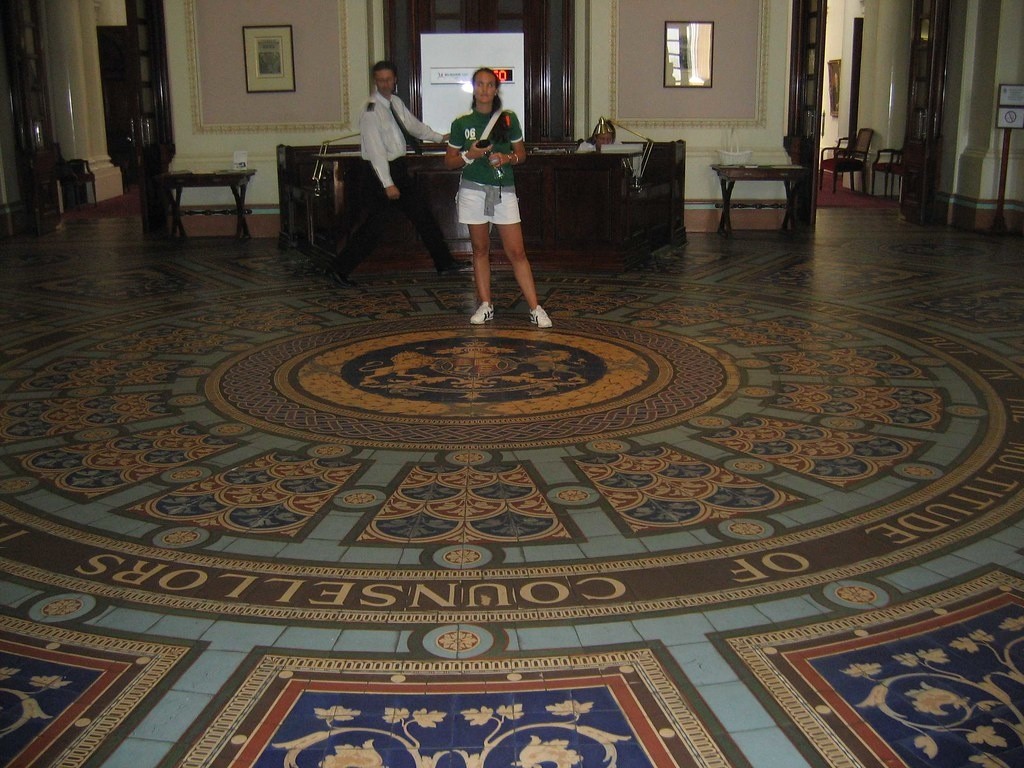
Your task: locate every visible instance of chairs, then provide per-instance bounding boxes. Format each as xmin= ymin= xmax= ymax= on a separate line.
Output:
xmin=872 ymin=148 xmax=903 ymax=201
xmin=64 ymin=158 xmax=99 ymax=214
xmin=819 ymin=127 xmax=873 ymax=196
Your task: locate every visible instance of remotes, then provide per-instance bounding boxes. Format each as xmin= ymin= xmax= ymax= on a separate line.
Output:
xmin=476 ymin=139 xmax=491 ymax=149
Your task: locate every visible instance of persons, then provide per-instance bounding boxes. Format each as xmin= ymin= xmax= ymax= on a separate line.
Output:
xmin=444 ymin=68 xmax=553 ymax=329
xmin=592 ymin=122 xmax=616 ymax=152
xmin=329 ymin=60 xmax=473 ymax=288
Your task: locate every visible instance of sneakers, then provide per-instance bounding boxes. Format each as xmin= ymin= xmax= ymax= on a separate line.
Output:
xmin=470 ymin=302 xmax=494 ymax=324
xmin=529 ymin=305 xmax=552 ymax=327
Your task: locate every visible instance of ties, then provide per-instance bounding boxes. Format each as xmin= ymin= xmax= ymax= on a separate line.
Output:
xmin=390 ymin=102 xmax=423 ymax=156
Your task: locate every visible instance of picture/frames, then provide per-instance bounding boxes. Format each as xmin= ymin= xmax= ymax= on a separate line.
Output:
xmin=828 ymin=60 xmax=841 ymax=117
xmin=242 ymin=25 xmax=295 ymax=93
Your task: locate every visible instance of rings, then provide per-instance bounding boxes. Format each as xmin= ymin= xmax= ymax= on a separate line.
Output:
xmin=484 ymin=152 xmax=486 ymax=154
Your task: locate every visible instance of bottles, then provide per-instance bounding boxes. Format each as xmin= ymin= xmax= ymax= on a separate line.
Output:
xmin=488 ymin=149 xmax=505 ymax=180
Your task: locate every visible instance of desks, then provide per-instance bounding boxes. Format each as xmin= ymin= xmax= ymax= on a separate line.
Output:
xmin=709 ymin=164 xmax=809 ymax=239
xmin=152 ymin=169 xmax=257 ymax=242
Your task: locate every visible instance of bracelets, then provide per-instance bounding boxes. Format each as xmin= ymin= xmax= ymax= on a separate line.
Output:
xmin=508 ymin=153 xmax=518 ymax=165
xmin=461 ymin=150 xmax=475 ymax=165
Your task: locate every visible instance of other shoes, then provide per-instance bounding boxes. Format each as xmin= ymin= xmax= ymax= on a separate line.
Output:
xmin=320 ymin=270 xmax=356 ymax=287
xmin=438 ymin=260 xmax=472 ymax=274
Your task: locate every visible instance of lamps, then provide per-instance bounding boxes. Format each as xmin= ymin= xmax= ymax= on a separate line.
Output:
xmin=592 ymin=117 xmax=654 ymax=192
